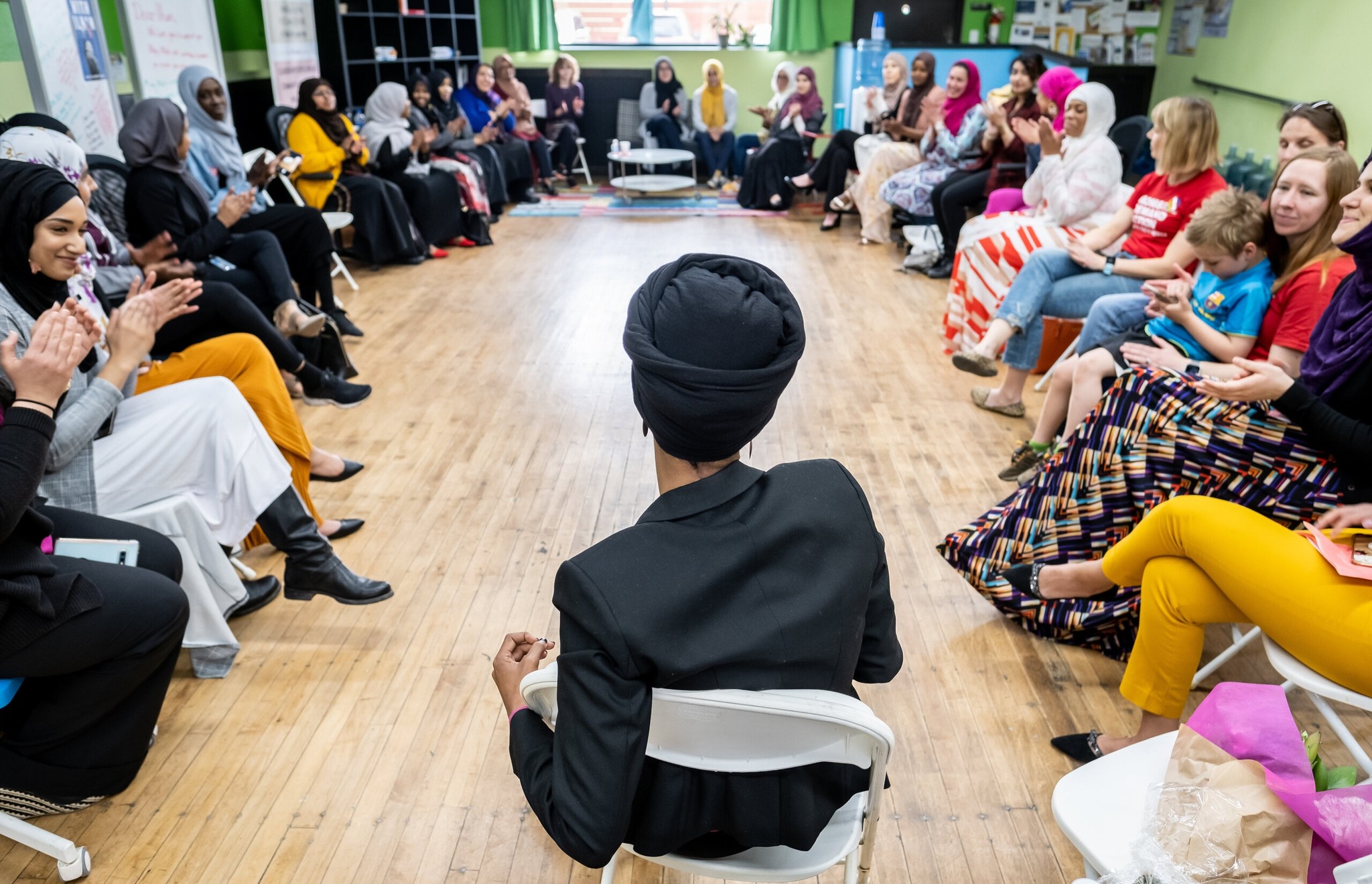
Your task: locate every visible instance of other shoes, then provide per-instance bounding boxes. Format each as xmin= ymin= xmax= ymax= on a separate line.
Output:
xmin=318 ymin=304 xmax=364 ymax=336
xmin=541 ymin=181 xmax=558 ymax=196
xmin=450 ymin=236 xmax=475 ymax=247
xmin=952 ymin=349 xmax=998 ymax=377
xmin=971 ymin=386 xmax=1026 ymax=417
xmin=1050 ymin=728 xmax=1105 ymax=764
xmin=707 ymin=175 xmax=730 ymax=189
xmin=999 ymin=563 xmax=1121 ymax=603
xmin=511 ymin=184 xmax=541 ymax=204
xmin=433 ymin=248 xmax=449 ymax=258
xmin=327 ymin=518 xmax=365 ymax=540
xmin=309 ymin=458 xmax=364 ymax=482
xmin=923 ymin=257 xmax=953 ymax=279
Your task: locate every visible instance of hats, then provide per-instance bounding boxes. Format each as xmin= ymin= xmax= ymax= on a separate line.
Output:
xmin=622 ymin=251 xmax=806 ymax=462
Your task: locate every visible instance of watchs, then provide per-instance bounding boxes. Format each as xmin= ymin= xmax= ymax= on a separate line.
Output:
xmin=1103 ymin=255 xmax=1116 ymax=276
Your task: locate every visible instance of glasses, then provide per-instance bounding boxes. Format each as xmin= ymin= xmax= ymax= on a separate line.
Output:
xmin=1292 ymin=100 xmax=1345 ymax=141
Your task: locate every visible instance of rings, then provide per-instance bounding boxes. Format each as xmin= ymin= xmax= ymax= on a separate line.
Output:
xmin=1145 ymin=359 xmax=1150 ymax=364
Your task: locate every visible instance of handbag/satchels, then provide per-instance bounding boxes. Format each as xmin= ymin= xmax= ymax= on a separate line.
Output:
xmin=1156 ymin=722 xmax=1313 ymax=884
xmin=902 ymin=221 xmax=944 ymax=268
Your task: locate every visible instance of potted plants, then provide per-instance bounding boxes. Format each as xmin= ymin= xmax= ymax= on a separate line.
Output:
xmin=708 ymin=1 xmax=756 ymax=50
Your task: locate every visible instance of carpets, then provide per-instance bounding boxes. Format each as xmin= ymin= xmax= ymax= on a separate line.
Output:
xmin=509 ymin=190 xmax=791 ymax=217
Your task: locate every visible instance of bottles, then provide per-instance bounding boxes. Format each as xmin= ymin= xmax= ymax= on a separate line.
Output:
xmin=610 ymin=139 xmax=619 ymax=159
xmin=1225 ymin=149 xmax=1260 ymax=189
xmin=1243 ymin=155 xmax=1275 ymax=202
xmin=855 ymin=38 xmax=890 ymax=89
xmin=1215 ymin=146 xmax=1243 ymax=180
xmin=353 ymin=108 xmax=365 ymax=129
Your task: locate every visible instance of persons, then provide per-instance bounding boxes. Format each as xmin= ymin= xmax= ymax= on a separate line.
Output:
xmin=492 ymin=252 xmax=903 ymax=869
xmin=0 ymin=64 xmax=394 ymax=820
xmin=284 ymin=53 xmax=558 ymax=265
xmin=637 ymin=55 xmax=688 ymax=174
xmin=691 ymin=59 xmax=738 ymax=189
xmin=544 ymin=57 xmax=585 ymax=187
xmin=721 ymin=60 xmax=823 ymax=210
xmin=784 ymin=52 xmax=1372 ymax=764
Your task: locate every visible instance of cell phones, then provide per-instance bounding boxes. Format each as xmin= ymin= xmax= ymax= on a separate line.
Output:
xmin=54 ymin=537 xmax=139 ymax=567
xmin=1145 ymin=283 xmax=1176 ymax=304
xmin=277 ymin=150 xmax=303 ymax=175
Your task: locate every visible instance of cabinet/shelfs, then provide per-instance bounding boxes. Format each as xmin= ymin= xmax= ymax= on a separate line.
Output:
xmin=607 ymin=147 xmax=703 ymax=207
xmin=312 ymin=0 xmax=484 ymax=113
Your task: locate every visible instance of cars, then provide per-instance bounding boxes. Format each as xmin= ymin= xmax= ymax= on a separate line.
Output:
xmin=614 ymin=7 xmax=700 ymax=45
xmin=554 ymin=8 xmax=592 ymax=44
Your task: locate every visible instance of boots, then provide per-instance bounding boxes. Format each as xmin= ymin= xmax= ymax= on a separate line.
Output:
xmin=227 ymin=575 xmax=282 ymax=621
xmin=255 ymin=485 xmax=395 ymax=605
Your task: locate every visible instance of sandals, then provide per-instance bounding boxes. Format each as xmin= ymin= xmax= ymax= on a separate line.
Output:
xmin=855 ymin=237 xmax=877 ymax=245
xmin=829 ymin=190 xmax=854 ymax=211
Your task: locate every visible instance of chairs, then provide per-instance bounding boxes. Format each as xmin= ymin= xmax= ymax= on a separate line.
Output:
xmin=607 ymin=96 xmax=694 ymax=149
xmin=239 ymin=148 xmax=361 ymax=292
xmin=1044 ymin=729 xmax=1222 ymax=884
xmin=1108 ymin=114 xmax=1152 ymax=183
xmin=512 ymin=665 xmax=899 ymax=884
xmin=528 ymin=96 xmax=595 ymax=189
xmin=1260 ymin=632 xmax=1372 ymax=791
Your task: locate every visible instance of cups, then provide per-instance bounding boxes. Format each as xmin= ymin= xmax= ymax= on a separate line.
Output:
xmin=871 ymin=11 xmax=886 ymax=39
xmin=620 ymin=141 xmax=631 ymax=154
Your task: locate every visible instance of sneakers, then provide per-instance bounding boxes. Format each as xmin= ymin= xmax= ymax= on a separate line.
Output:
xmin=302 ymin=366 xmax=372 ymax=409
xmin=997 ymin=440 xmax=1061 ymax=488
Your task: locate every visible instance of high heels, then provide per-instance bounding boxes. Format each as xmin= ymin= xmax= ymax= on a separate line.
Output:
xmin=274 ymin=299 xmax=326 ymax=340
xmin=778 ymin=176 xmax=818 ymax=196
xmin=820 ymin=213 xmax=841 ymax=231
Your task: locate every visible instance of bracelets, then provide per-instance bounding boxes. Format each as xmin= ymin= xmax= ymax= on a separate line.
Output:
xmin=998 ymin=123 xmax=1010 ymax=133
xmin=983 ymin=131 xmax=999 ymax=140
xmin=12 ymin=398 xmax=55 ymax=415
xmin=1185 ymin=358 xmax=1201 ymax=377
